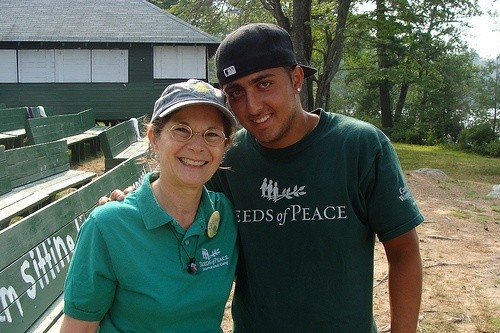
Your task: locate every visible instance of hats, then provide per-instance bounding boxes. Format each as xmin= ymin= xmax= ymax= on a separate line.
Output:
xmin=216 ymin=21 xmax=318 ymax=87
xmin=151 ymin=79 xmax=237 ymax=138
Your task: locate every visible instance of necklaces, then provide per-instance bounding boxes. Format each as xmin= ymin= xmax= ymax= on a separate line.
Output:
xmin=164 ymin=220 xmax=198 ymax=274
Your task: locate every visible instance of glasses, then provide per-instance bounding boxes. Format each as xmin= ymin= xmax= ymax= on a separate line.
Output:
xmin=160 ymin=123 xmax=229 ymax=147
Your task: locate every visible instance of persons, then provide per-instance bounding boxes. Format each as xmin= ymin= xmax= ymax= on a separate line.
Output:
xmin=61 ymin=79 xmax=237 ymax=333
xmin=101 ymin=22 xmax=422 ymax=333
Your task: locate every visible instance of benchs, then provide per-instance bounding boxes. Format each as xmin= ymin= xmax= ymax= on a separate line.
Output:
xmin=0 ymin=101 xmax=156 ymax=333
